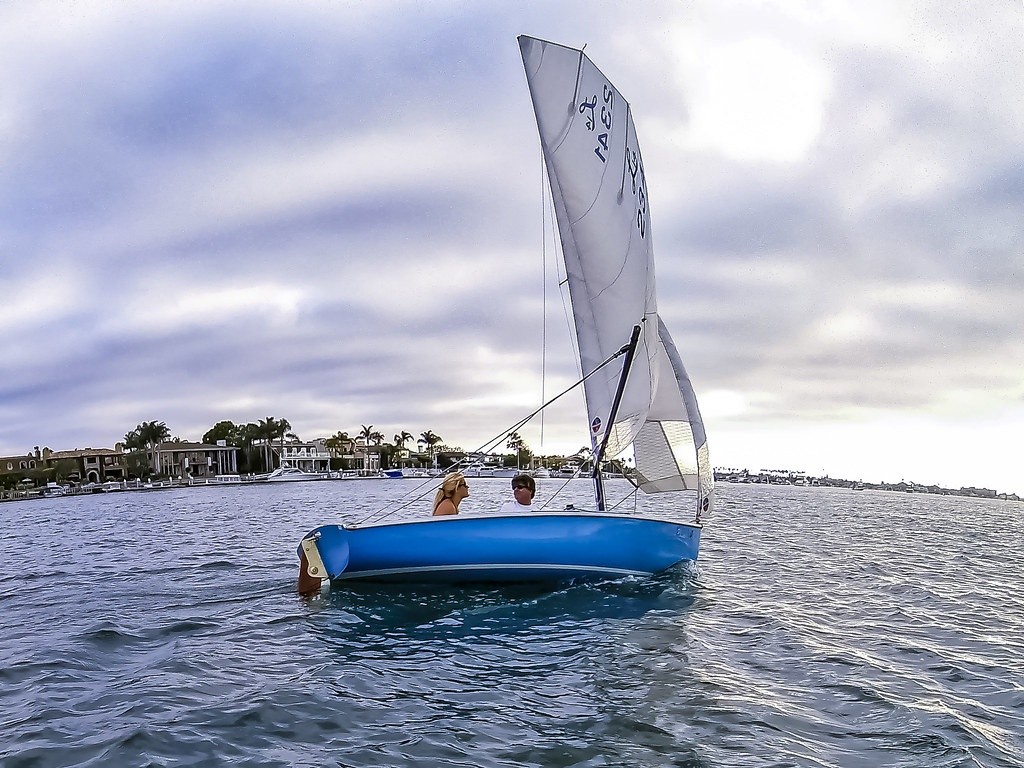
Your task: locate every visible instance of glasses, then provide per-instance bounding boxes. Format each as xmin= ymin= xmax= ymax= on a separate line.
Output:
xmin=512 ymin=485 xmax=527 ymax=490
xmin=460 ymin=481 xmax=466 ymax=487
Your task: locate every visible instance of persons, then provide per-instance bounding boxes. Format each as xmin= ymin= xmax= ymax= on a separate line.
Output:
xmin=500 ymin=474 xmax=541 ymax=512
xmin=433 ymin=472 xmax=469 ymax=516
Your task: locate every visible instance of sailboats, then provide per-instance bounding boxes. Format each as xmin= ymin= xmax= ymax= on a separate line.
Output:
xmin=296 ymin=34 xmax=717 ymax=601
